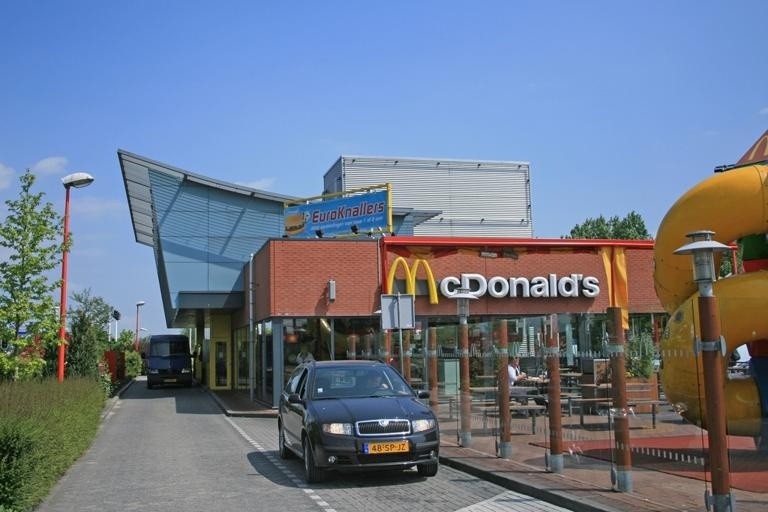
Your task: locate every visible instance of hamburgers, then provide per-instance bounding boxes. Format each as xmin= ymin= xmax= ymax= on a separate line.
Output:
xmin=285 ymin=215 xmax=305 ymax=234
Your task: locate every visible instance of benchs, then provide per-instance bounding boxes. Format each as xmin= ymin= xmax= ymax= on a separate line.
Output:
xmin=449 ymin=394 xmax=667 ymax=441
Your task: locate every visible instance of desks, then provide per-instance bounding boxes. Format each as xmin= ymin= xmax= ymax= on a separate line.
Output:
xmin=459 ymin=374 xmax=656 ymax=394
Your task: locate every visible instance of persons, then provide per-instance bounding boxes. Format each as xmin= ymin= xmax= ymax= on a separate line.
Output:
xmin=353 ymin=371 xmax=390 ymax=397
xmin=296 ymin=344 xmax=315 ymax=366
xmin=505 ymin=352 xmax=549 ymax=411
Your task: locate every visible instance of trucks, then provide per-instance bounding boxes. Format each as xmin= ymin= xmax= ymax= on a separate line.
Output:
xmin=141 ymin=334 xmax=200 ymax=391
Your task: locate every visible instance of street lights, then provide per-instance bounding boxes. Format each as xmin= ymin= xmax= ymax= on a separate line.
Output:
xmin=673 ymin=229 xmax=737 ymax=511
xmin=446 ymin=287 xmax=480 ymax=447
xmin=135 ymin=300 xmax=145 ymax=354
xmin=56 ymin=171 xmax=95 ymax=384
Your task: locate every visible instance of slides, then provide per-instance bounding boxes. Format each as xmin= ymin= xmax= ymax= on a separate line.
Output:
xmin=653 ymin=166 xmax=768 ymax=456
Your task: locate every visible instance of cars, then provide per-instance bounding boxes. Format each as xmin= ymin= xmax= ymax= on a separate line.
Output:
xmin=274 ymin=357 xmax=441 ymax=485
